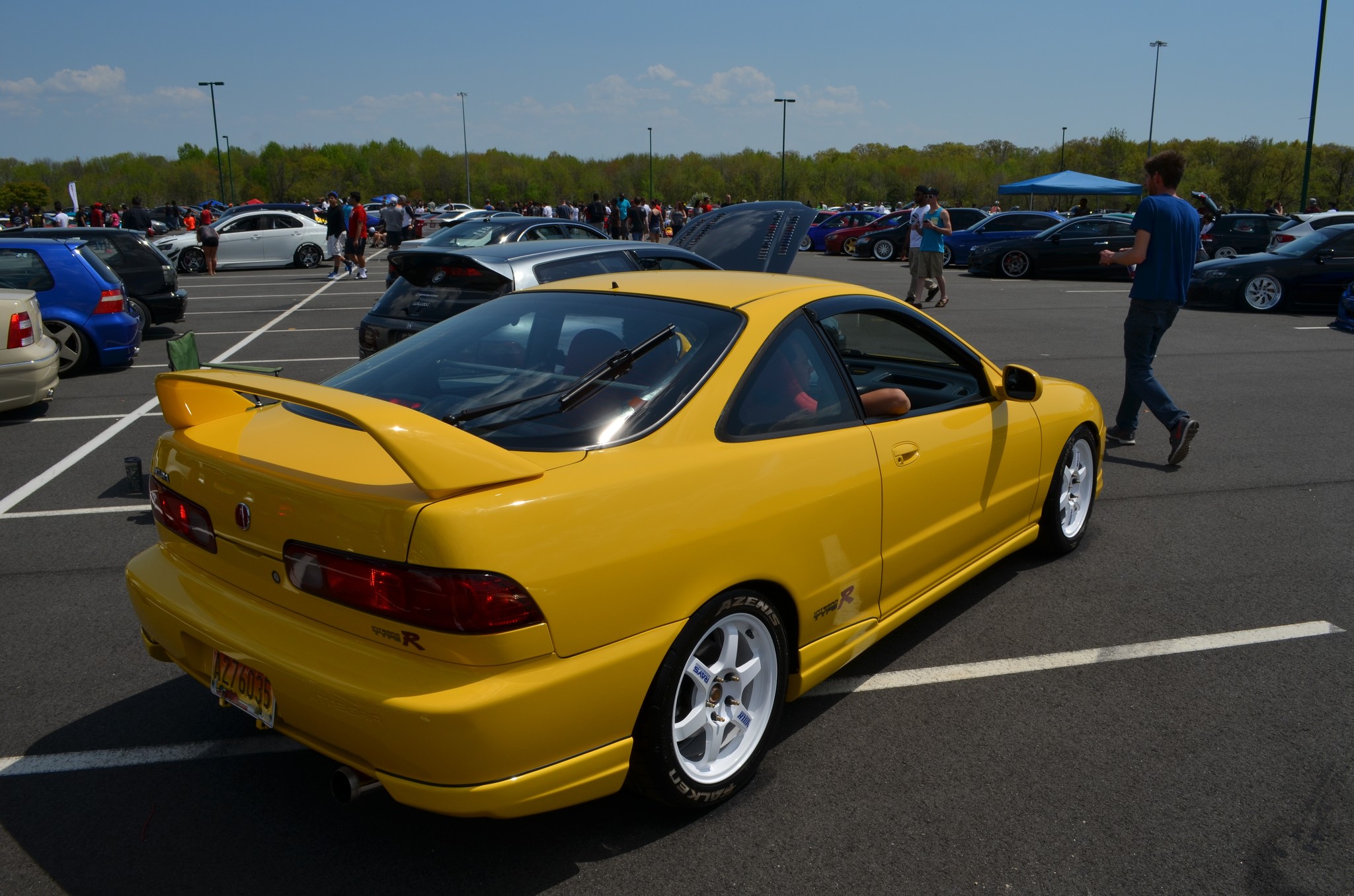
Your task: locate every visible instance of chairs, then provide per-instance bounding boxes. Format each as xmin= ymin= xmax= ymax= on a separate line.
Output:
xmin=740 ymin=354 xmax=817 ymax=433
xmin=567 ymin=330 xmax=630 ymax=407
xmin=856 ymin=214 xmax=866 ymax=227
xmin=167 ymin=330 xmax=282 ymax=405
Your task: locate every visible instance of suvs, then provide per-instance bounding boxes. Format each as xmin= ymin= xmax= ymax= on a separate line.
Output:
xmin=1189 ymin=190 xmax=1292 ymax=260
xmin=1265 ymin=209 xmax=1354 ymax=253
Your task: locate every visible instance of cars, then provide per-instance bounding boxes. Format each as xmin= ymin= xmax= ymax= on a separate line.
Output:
xmin=1180 ymin=223 xmax=1354 ymax=313
xmin=0 ymin=223 xmax=188 ymax=338
xmin=399 ymin=217 xmax=547 ymax=250
xmin=123 ymin=271 xmax=1106 ymax=823
xmin=0 ymin=237 xmax=142 ymax=379
xmin=365 ymin=213 xmax=381 ymax=227
xmin=415 ymin=212 xmax=446 ymax=239
xmin=386 ymin=216 xmax=614 ymax=291
xmin=422 ymin=209 xmax=487 ymax=239
xmin=151 ymin=210 xmax=333 ymax=273
xmin=941 ymin=211 xmax=1099 ymax=267
xmin=435 ymin=203 xmax=476 ymax=212
xmin=967 ymin=214 xmax=1137 ymax=279
xmin=439 ymin=210 xmax=524 ymax=229
xmin=767 ymin=210 xmax=869 ymax=245
xmin=358 ymin=200 xmax=820 ymax=376
xmin=663 ymin=203 xmax=722 ymax=238
xmin=0 ymin=201 xmax=329 ymax=247
xmin=824 ymin=208 xmax=915 ymax=256
xmin=852 ymin=206 xmax=991 ymax=261
xmin=338 ymin=198 xmax=383 ymax=218
xmin=0 ymin=287 xmax=60 ymax=412
xmin=1058 ymin=205 xmax=1135 ymax=220
xmin=1334 ymin=280 xmax=1354 ymax=333
xmin=797 ymin=211 xmax=899 ymax=252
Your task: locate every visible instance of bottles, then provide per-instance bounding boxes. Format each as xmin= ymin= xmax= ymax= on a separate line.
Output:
xmin=1126 ymin=264 xmax=1137 ymax=279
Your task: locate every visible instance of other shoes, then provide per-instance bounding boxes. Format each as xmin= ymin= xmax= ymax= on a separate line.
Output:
xmin=369 ymin=244 xmax=389 ymax=249
xmin=326 ymin=261 xmax=368 ymax=280
xmin=904 ymin=287 xmax=949 ymax=309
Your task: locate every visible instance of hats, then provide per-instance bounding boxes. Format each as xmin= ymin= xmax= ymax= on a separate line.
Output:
xmin=326 ymin=191 xmax=339 ymax=198
xmin=390 ymin=197 xmax=397 ymax=203
xmin=923 ymin=187 xmax=939 ymax=195
xmin=914 ymin=185 xmax=928 ymax=195
xmin=399 ymin=195 xmax=409 ymax=203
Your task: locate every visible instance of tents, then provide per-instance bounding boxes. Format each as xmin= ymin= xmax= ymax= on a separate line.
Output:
xmin=996 ymin=170 xmax=1143 ymax=214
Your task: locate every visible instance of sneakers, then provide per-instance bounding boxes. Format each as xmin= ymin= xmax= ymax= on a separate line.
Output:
xmin=1106 ymin=426 xmax=1136 ymax=444
xmin=1167 ymin=416 xmax=1199 ymax=466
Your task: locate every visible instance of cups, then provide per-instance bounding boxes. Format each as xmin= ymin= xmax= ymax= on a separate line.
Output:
xmin=124 ymin=457 xmax=144 ymax=492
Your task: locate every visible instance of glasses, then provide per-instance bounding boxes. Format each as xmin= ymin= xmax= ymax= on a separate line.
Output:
xmin=398 ymin=200 xmax=400 ymax=202
xmin=913 ymin=194 xmax=921 ymax=196
xmin=924 ymin=195 xmax=936 ymax=199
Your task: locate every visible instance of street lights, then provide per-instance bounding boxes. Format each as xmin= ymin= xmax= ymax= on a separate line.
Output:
xmin=1057 ymin=127 xmax=1068 ymax=213
xmin=1147 ymin=40 xmax=1169 ymax=161
xmin=647 ymin=127 xmax=652 ymax=201
xmin=457 ymin=92 xmax=471 ymax=207
xmin=198 ymin=81 xmax=226 ymax=207
xmin=223 ymin=135 xmax=235 ymax=204
xmin=774 ymin=99 xmax=796 ymax=200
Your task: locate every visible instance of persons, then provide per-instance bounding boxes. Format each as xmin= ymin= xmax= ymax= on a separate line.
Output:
xmin=1274 ymin=201 xmax=1283 ymax=216
xmin=1326 ymin=201 xmax=1339 ymax=212
xmin=1200 ymin=212 xmax=1214 ymax=235
xmin=1100 ymin=207 xmax=1106 ymax=214
xmin=0 ymin=180 xmax=940 ymax=435
xmin=911 ymin=187 xmax=952 ymax=309
xmin=955 ymin=201 xmax=963 ymax=208
xmin=1303 ymin=198 xmax=1321 ymax=214
xmin=1230 ymin=206 xmax=1237 ymax=214
xmin=1073 ymin=197 xmax=1091 ymax=218
xmin=1253 ymin=210 xmax=1260 ymax=214
xmin=1099 ymin=150 xmax=1200 ymax=465
xmin=990 ymin=200 xmax=1001 ymax=214
xmin=1121 ymin=202 xmax=1133 ymax=215
xmin=1264 ymin=199 xmax=1277 ymax=214
